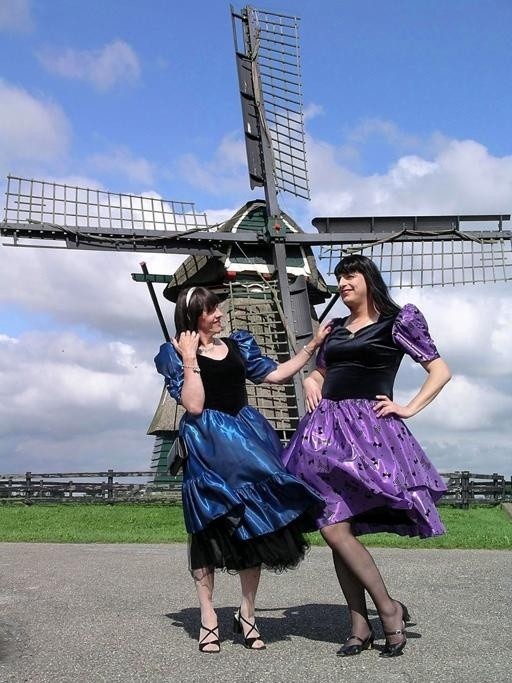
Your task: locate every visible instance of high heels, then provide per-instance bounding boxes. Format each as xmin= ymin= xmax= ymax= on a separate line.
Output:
xmin=234 ymin=607 xmax=266 ymax=649
xmin=199 ymin=622 xmax=220 ymax=652
xmin=337 ymin=631 xmax=375 ymax=655
xmin=379 ymin=600 xmax=410 ymax=655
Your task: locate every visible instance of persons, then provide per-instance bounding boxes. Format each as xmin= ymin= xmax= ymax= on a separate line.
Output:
xmin=276 ymin=253 xmax=453 ymax=656
xmin=152 ymin=284 xmax=334 ymax=652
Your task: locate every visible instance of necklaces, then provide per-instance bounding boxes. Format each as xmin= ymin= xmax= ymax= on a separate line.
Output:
xmin=198 ymin=343 xmax=216 ymax=350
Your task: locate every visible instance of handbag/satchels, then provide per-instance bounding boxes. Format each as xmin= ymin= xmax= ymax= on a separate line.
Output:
xmin=167 ymin=437 xmax=188 ymax=476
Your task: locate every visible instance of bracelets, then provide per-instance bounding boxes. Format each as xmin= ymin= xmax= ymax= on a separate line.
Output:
xmin=180 ymin=363 xmax=201 ymax=373
xmin=302 ymin=344 xmax=314 ymax=357
